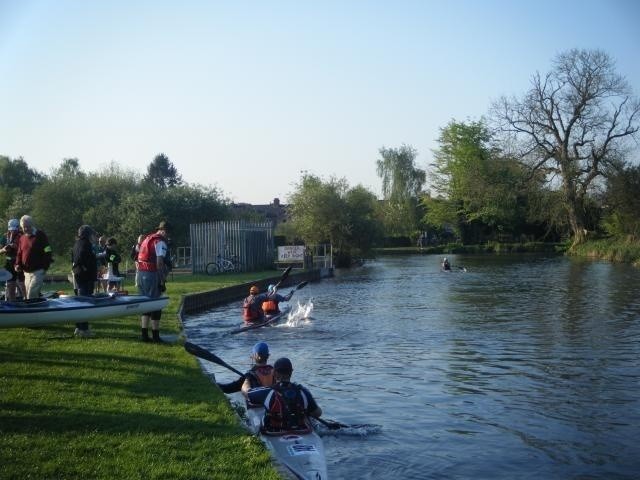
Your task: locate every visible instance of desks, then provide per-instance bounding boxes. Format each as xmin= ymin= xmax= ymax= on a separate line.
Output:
xmin=67 ymin=274 xmax=125 ymax=295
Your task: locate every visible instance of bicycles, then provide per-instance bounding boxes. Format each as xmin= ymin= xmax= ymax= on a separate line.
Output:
xmin=205 ymin=254 xmax=240 ymax=276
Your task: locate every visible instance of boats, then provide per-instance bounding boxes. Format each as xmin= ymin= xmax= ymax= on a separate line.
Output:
xmin=230 ymin=304 xmax=293 ymax=335
xmin=0 ymin=290 xmax=171 ymax=329
xmin=244 ymin=385 xmax=329 ymax=480
xmin=442 ymin=267 xmax=467 ymax=274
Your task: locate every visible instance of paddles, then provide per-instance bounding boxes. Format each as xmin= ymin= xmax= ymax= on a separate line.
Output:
xmin=452 ymin=266 xmax=463 ymax=270
xmin=289 ymin=281 xmax=307 ymax=293
xmin=183 ymin=340 xmax=342 ymax=429
xmin=265 ymin=265 xmax=292 ymax=295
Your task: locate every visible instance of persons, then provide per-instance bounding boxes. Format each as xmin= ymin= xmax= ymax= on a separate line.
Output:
xmin=93 ymin=234 xmax=108 ymax=294
xmin=132 ymin=218 xmax=175 ymax=346
xmin=238 ymin=341 xmax=277 ymax=401
xmin=262 ymin=283 xmax=295 ymax=318
xmin=0 ymin=218 xmax=28 ymax=301
xmin=160 ymin=236 xmax=173 ymax=293
xmin=68 ymin=222 xmax=106 ymax=339
xmin=103 ymin=237 xmax=124 ymax=293
xmin=242 ymin=284 xmax=279 ymax=326
xmin=14 ymin=216 xmax=53 ymax=301
xmin=132 ymin=233 xmax=146 ymax=287
xmin=245 ymin=355 xmax=323 ymax=434
xmin=441 ymin=257 xmax=450 ymax=271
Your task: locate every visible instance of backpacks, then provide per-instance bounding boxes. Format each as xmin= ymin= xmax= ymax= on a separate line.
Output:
xmin=265 ymin=383 xmax=312 ymax=432
xmin=243 ymin=297 xmax=255 ymax=321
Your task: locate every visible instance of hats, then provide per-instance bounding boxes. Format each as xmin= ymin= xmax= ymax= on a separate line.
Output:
xmin=249 ymin=285 xmax=259 ymax=293
xmin=274 ymin=357 xmax=292 ymax=370
xmin=252 ymin=342 xmax=269 ymax=355
xmin=7 ymin=218 xmax=19 ymax=231
xmin=154 ymin=221 xmax=169 ymax=230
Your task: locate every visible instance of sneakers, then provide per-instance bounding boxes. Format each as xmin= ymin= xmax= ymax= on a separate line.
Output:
xmin=74 ymin=328 xmax=96 ymax=337
xmin=141 ymin=336 xmax=165 ymax=342
